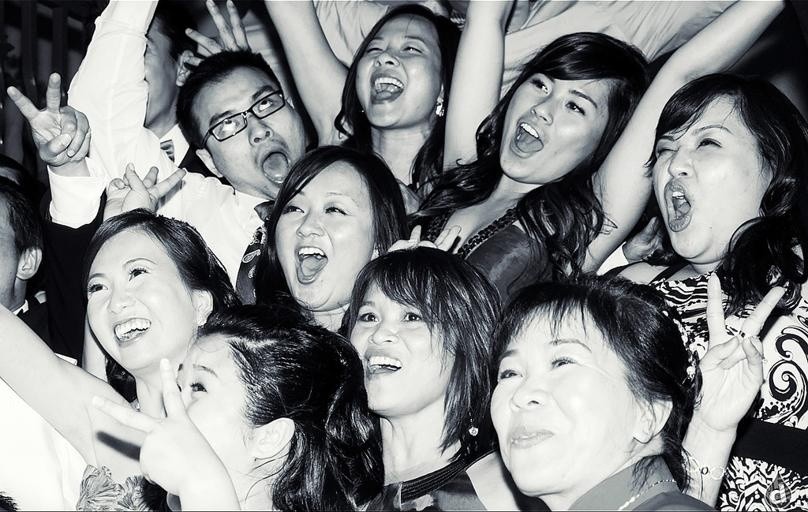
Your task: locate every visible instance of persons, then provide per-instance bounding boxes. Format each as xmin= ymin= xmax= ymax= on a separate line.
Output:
xmin=1 ymin=1 xmax=808 ymax=512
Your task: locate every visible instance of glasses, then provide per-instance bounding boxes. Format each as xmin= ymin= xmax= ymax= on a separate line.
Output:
xmin=201 ymin=90 xmax=285 ymax=144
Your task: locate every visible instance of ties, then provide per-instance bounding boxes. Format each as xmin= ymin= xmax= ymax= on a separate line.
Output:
xmin=234 ymin=202 xmax=272 ymax=303
